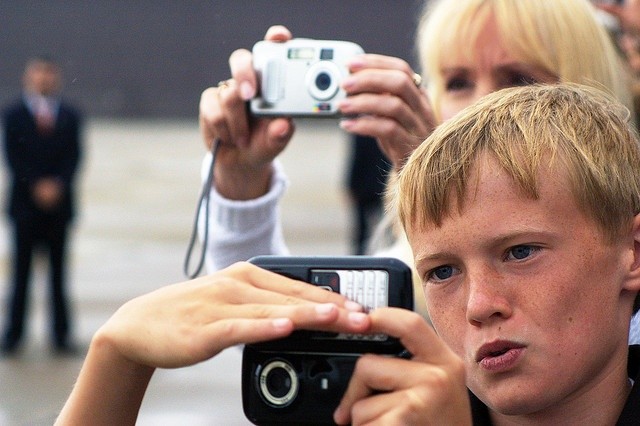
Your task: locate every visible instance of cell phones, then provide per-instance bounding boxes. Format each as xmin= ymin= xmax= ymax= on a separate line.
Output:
xmin=241 ymin=255 xmax=415 ymax=425
xmin=251 ymin=38 xmax=366 ymax=117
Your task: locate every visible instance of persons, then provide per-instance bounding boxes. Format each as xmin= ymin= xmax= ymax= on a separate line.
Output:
xmin=53 ymin=82 xmax=640 ymax=426
xmin=198 ymin=0 xmax=633 ymax=275
xmin=344 ymin=115 xmax=394 ymax=255
xmin=0 ymin=53 xmax=89 ymax=358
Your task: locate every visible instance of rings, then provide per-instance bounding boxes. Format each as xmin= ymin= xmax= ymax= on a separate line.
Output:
xmin=411 ymin=70 xmax=422 ymax=87
